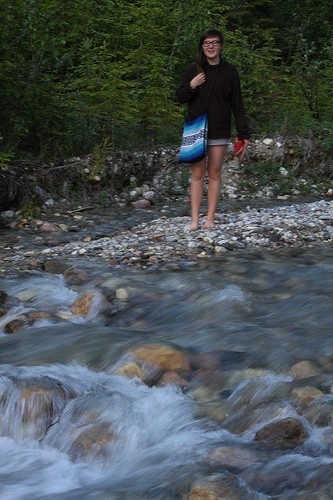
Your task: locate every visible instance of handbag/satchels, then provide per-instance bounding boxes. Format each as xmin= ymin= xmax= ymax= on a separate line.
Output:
xmin=178 ymin=113 xmax=208 ymax=165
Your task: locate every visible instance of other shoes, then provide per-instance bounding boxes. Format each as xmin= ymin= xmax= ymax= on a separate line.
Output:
xmin=230 ymin=134 xmax=248 ymax=161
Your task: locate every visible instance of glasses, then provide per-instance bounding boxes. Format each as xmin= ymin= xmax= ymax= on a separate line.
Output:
xmin=202 ymin=40 xmax=220 ymax=47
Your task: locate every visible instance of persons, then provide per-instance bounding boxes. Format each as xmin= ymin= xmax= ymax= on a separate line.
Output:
xmin=175 ymin=29 xmax=251 ymax=232
